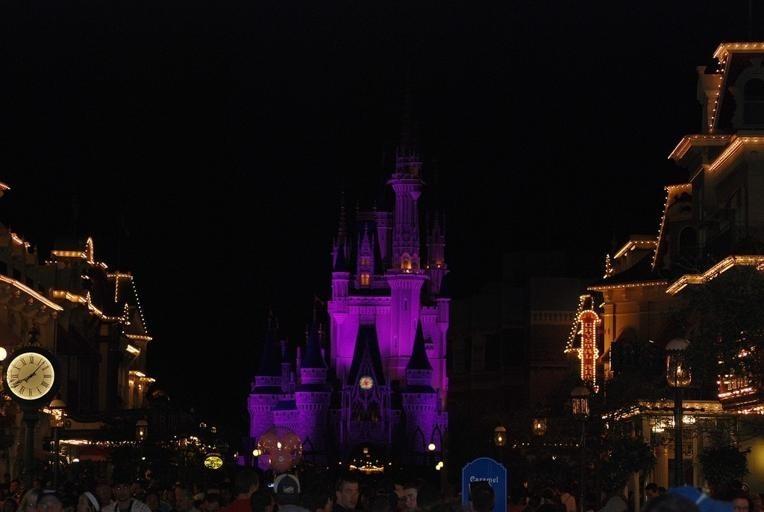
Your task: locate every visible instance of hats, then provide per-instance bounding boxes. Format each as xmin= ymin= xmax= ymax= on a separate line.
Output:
xmin=273 ymin=474 xmax=301 ymax=495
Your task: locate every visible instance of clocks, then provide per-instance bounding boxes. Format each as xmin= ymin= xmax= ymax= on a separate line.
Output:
xmin=3 ymin=346 xmax=61 ymax=409
xmin=358 ymin=375 xmax=374 ymax=390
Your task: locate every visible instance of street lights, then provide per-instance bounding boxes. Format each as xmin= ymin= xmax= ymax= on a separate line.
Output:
xmin=134 ymin=417 xmax=149 ymax=482
xmin=490 ymin=381 xmax=591 ymax=512
xmin=663 ymin=338 xmax=694 ymax=488
xmin=49 ymin=398 xmax=66 ymax=490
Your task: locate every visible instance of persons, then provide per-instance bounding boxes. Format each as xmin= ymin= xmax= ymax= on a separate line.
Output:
xmin=0 ymin=454 xmax=764 ymax=512
